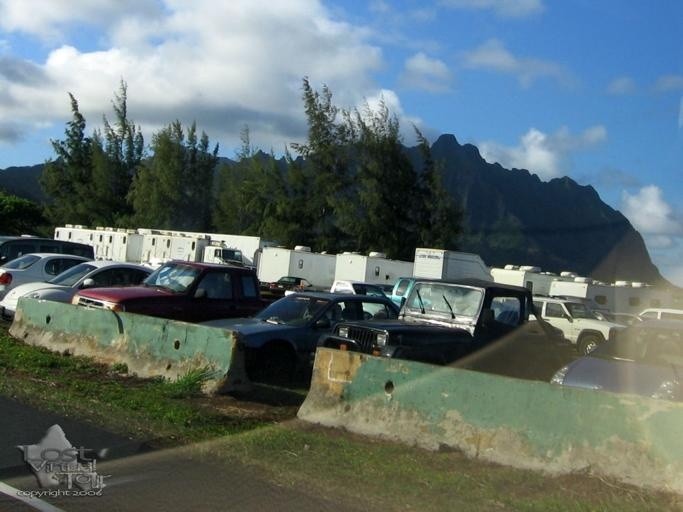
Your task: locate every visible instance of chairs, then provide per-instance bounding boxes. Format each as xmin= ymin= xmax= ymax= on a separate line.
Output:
xmin=497 ymin=310 xmax=518 ymax=325
xmin=302 ymin=302 xmax=342 ymax=327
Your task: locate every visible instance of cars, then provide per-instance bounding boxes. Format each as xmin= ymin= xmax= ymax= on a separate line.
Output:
xmin=0 ymin=224 xmax=683 ymax=402
